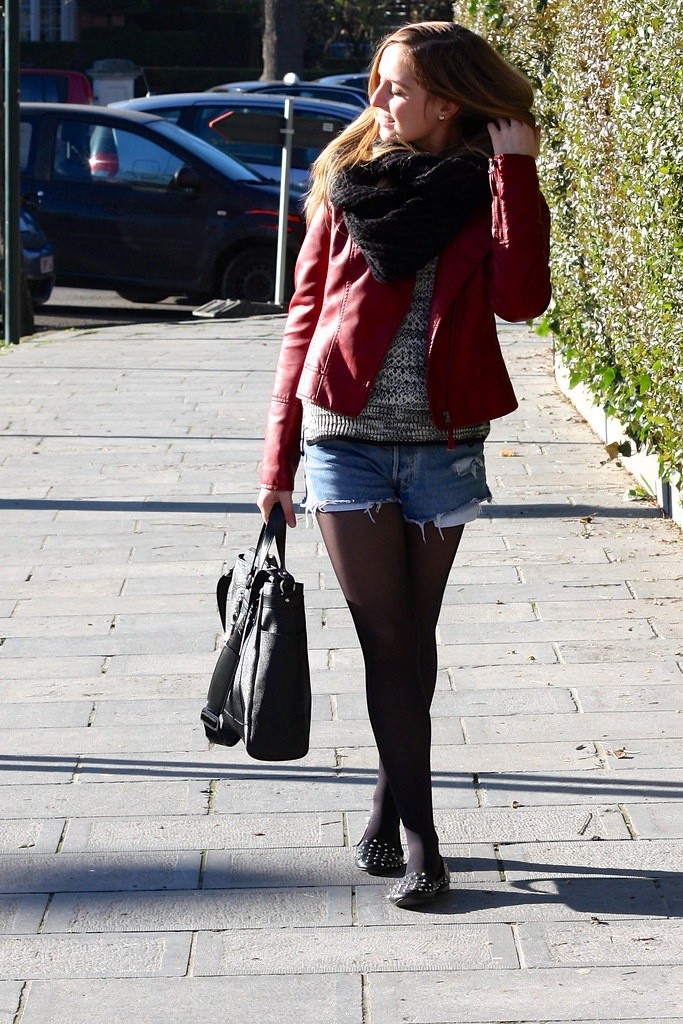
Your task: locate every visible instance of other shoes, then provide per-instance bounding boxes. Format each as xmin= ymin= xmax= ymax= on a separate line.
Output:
xmin=389 ymin=859 xmax=450 ymax=905
xmin=355 ymin=816 xmax=404 ymax=870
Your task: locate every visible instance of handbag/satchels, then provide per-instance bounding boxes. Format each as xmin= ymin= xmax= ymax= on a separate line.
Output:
xmin=200 ymin=502 xmax=312 ymax=762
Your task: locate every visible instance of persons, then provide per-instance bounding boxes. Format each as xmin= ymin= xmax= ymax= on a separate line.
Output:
xmin=256 ymin=20 xmax=553 ymax=908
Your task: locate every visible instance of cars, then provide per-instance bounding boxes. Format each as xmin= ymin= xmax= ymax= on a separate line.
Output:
xmin=202 ymin=71 xmax=371 ymax=111
xmin=21 ymin=68 xmax=93 ymax=104
xmin=90 ymin=90 xmax=363 ymax=187
xmin=20 ymin=101 xmax=309 ymax=306
